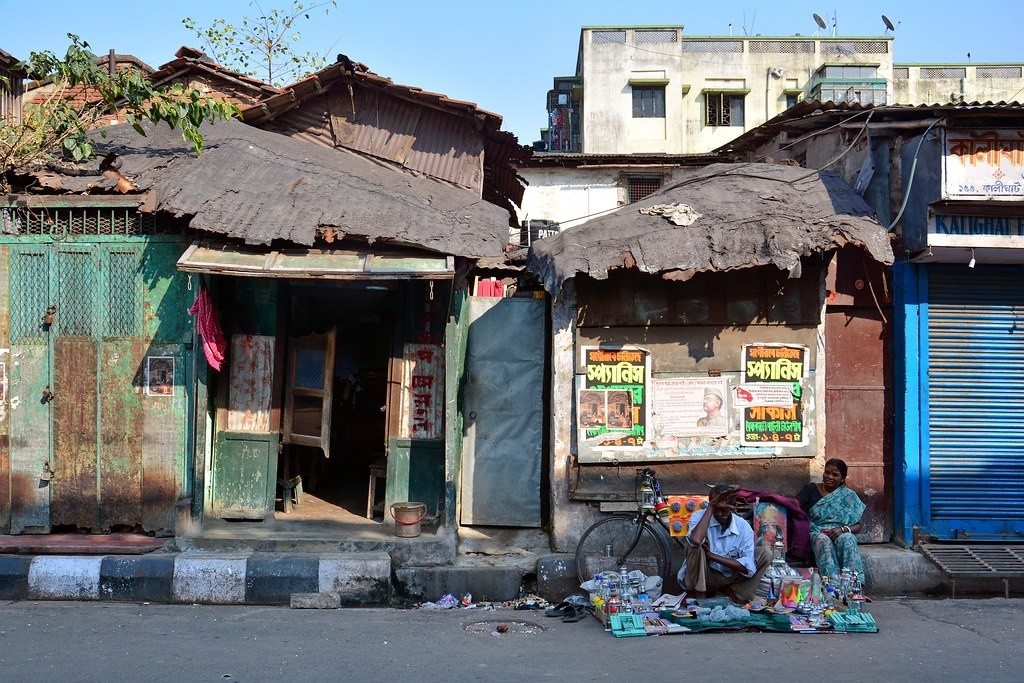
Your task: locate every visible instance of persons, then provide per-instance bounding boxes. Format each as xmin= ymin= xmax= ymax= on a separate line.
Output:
xmin=697 ymin=386 xmax=726 ymax=427
xmin=681 ymin=483 xmax=774 ymax=605
xmin=793 ymin=457 xmax=867 ymax=603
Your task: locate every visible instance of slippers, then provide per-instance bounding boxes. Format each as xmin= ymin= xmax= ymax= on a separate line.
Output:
xmin=545 ymin=601 xmax=587 ymax=621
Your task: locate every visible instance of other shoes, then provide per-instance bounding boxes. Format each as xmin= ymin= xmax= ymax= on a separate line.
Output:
xmin=728 ymin=592 xmax=750 ymax=607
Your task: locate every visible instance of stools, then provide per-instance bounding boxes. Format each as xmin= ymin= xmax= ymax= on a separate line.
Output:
xmin=367 ymin=451 xmax=387 ymax=521
xmin=277 ymin=432 xmax=303 ymax=513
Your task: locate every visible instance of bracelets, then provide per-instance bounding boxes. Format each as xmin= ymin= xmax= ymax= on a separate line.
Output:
xmin=844 ymin=526 xmax=851 ymax=533
xmin=840 ymin=526 xmax=845 ymax=533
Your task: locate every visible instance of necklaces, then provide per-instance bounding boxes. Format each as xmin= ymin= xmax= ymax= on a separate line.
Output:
xmin=823 ymin=483 xmax=829 ymax=493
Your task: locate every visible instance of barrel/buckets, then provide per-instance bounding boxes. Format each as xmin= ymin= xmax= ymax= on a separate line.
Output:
xmin=390 ymin=502 xmax=426 ymax=537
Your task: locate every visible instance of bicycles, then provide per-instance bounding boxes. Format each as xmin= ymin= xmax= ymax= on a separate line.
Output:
xmin=573 ymin=467 xmax=817 ymax=594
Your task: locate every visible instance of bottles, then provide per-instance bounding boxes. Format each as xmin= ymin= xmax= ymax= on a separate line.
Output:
xmin=810 ymin=568 xmax=820 ymax=605
xmin=782 ymin=576 xmax=803 ymax=608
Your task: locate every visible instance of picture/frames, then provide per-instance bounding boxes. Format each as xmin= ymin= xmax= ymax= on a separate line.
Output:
xmin=147 ymin=355 xmax=175 ymax=397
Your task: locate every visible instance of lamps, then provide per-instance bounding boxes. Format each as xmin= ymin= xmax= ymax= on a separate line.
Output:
xmin=967 ymin=248 xmax=977 ymax=268
